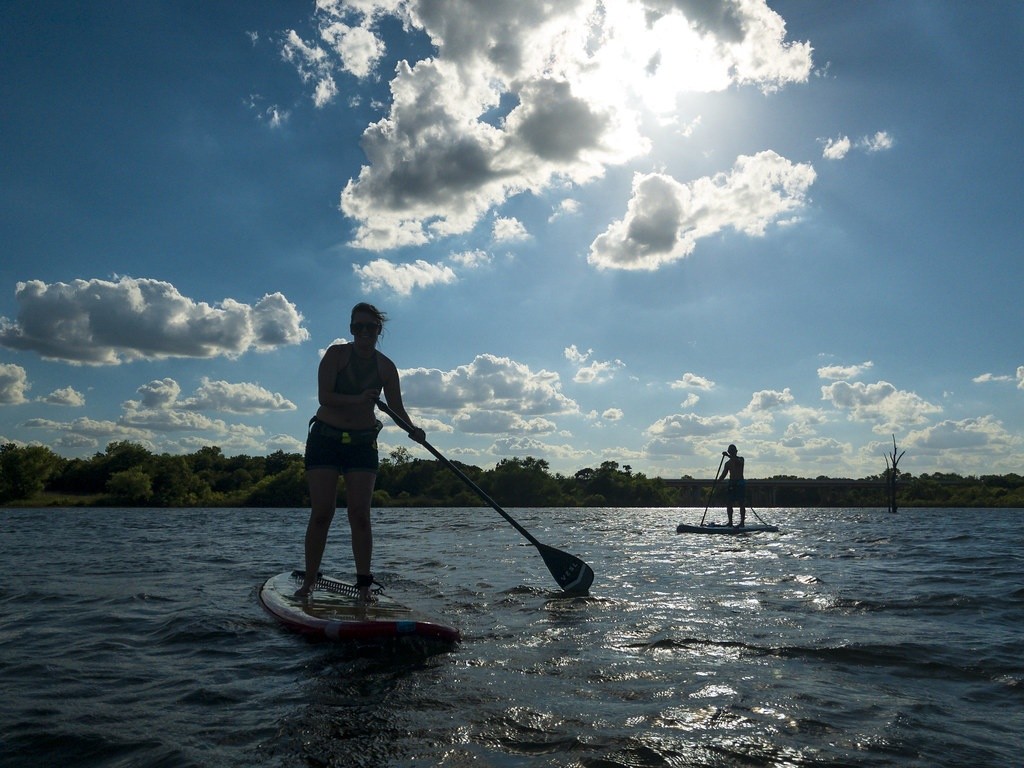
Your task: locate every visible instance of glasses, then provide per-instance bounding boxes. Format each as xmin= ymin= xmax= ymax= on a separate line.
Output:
xmin=352 ymin=322 xmax=381 ymax=330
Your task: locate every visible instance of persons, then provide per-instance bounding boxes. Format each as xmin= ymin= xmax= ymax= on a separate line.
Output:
xmin=293 ymin=302 xmax=426 ymax=604
xmin=714 ymin=444 xmax=746 ymax=527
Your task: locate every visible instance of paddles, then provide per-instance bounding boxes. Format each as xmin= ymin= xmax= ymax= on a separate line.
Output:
xmin=368 ymin=393 xmax=596 ymax=598
xmin=698 ymin=454 xmax=725 ymax=526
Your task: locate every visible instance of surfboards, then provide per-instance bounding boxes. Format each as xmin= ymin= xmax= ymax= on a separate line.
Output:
xmin=258 ymin=568 xmax=459 ymax=663
xmin=676 ymin=522 xmax=777 ymax=534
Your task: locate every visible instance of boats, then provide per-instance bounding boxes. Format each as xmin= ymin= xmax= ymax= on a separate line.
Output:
xmin=260 ymin=567 xmax=464 ymax=645
xmin=676 ymin=520 xmax=779 ymax=534
